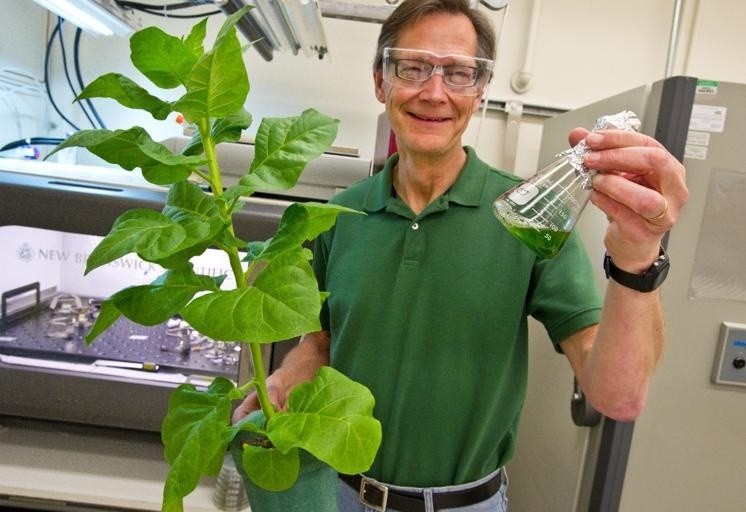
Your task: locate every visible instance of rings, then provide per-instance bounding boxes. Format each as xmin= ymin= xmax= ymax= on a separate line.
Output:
xmin=648 ymin=195 xmax=670 ymax=225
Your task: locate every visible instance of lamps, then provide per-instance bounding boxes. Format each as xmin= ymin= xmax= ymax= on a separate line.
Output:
xmin=34 ymin=0 xmax=142 ymax=40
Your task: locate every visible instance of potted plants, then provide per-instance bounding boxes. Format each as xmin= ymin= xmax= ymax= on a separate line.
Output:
xmin=44 ymin=5 xmax=399 ymax=511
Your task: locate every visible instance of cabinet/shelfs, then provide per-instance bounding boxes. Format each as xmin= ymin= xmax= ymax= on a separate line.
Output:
xmin=0 ymin=155 xmax=297 ymax=434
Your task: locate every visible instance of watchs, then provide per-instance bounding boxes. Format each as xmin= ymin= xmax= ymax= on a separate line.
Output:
xmin=604 ymin=245 xmax=669 ymax=295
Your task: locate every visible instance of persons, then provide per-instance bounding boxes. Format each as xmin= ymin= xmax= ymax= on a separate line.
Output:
xmin=229 ymin=0 xmax=692 ymax=512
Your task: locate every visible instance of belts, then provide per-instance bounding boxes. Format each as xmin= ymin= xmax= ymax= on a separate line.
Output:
xmin=338 ymin=472 xmax=501 ymax=512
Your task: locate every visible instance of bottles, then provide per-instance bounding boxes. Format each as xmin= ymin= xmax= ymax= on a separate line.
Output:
xmin=492 ymin=110 xmax=641 ymax=260
xmin=213 ymin=454 xmax=249 ymax=511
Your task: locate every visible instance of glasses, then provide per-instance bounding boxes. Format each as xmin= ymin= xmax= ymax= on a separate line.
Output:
xmin=383 ymin=48 xmax=495 ymax=97
